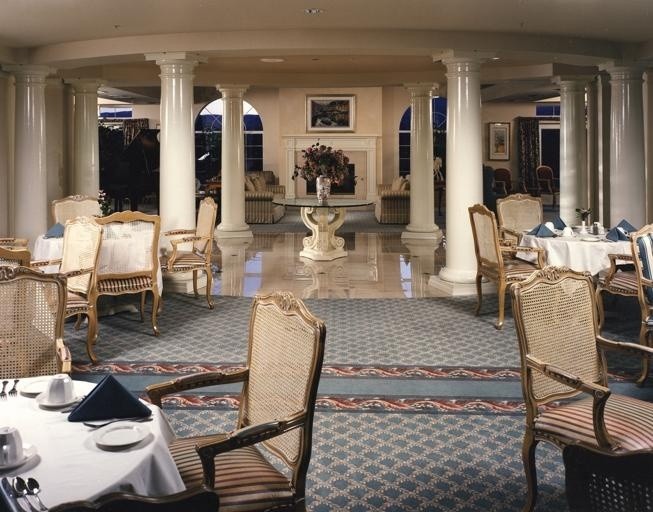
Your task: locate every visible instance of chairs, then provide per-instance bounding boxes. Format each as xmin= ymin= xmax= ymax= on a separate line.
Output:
xmin=561 ymin=437 xmax=651 ymax=510
xmin=50 ymin=485 xmax=221 ymax=511
xmin=507 ymin=263 xmax=653 ymax=511
xmin=148 ymin=290 xmax=327 ymax=512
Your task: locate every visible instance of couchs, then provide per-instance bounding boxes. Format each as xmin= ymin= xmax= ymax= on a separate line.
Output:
xmin=373 ymin=176 xmax=411 ymax=224
xmin=244 ymin=169 xmax=286 ymax=223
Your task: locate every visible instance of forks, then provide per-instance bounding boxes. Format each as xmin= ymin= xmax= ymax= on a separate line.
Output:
xmin=82 ymin=417 xmax=153 ymax=429
xmin=7 ymin=380 xmax=18 ymax=395
xmin=0 ymin=380 xmax=8 ymax=398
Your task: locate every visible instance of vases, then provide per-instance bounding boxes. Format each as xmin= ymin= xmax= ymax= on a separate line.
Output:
xmin=315 ymin=174 xmax=332 ymax=200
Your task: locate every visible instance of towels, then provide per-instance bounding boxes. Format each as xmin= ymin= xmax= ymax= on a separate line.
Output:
xmin=67 ymin=374 xmax=152 ymax=421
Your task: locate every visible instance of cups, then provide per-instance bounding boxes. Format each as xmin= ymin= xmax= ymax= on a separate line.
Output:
xmin=545 ymin=221 xmax=558 ymax=233
xmin=617 ymin=227 xmax=628 ymax=236
xmin=561 ymin=226 xmax=572 ymax=236
xmin=589 ymin=222 xmax=604 ymax=235
xmin=46 ymin=372 xmax=75 ymax=404
xmin=0 ymin=427 xmax=24 ymax=467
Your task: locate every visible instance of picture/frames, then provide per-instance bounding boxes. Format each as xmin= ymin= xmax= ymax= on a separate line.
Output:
xmin=304 ymin=93 xmax=357 ymax=133
xmin=488 ymin=121 xmax=510 ymax=161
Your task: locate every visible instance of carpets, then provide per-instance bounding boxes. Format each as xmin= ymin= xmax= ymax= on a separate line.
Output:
xmin=54 ymin=294 xmax=653 ymax=511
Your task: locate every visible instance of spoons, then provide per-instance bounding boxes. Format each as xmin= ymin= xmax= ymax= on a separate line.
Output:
xmin=12 ymin=476 xmax=39 ymax=512
xmin=24 ymin=477 xmax=47 ymax=510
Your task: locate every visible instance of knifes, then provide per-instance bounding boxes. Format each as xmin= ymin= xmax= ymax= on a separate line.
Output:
xmin=1 ymin=477 xmax=26 ymax=512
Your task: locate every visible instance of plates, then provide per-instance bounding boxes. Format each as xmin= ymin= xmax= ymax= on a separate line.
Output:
xmin=13 ymin=375 xmax=53 ymax=395
xmin=0 ymin=441 xmax=38 ymax=472
xmin=579 ymin=237 xmax=600 ymax=242
xmin=91 ymin=421 xmax=151 ymax=448
xmin=33 ymin=391 xmax=80 ymax=408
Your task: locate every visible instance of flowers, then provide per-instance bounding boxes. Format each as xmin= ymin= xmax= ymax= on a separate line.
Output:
xmin=290 ymin=139 xmax=351 ymax=184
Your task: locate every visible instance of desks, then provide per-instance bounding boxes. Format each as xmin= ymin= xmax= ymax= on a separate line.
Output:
xmin=271 ymin=197 xmax=373 ymax=262
